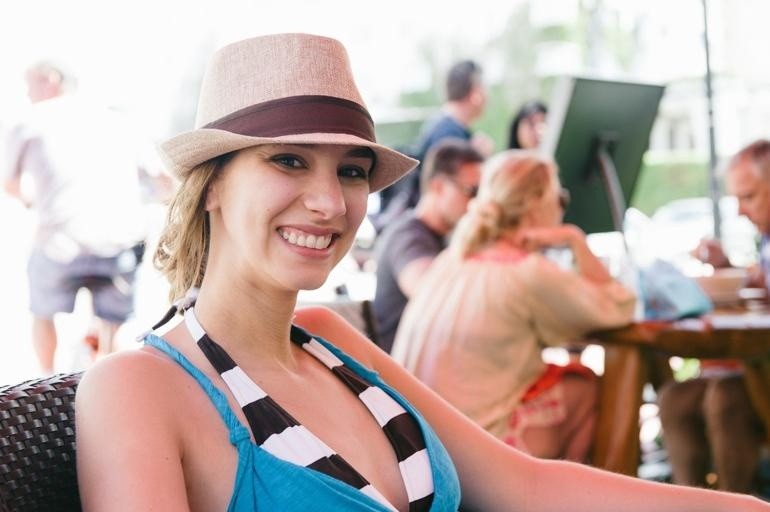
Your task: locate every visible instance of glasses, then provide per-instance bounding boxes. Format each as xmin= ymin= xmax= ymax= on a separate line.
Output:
xmin=446 ymin=174 xmax=479 ymax=200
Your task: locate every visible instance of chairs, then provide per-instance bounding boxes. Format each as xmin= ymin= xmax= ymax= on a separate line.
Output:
xmin=0 ymin=370 xmax=84 ymax=512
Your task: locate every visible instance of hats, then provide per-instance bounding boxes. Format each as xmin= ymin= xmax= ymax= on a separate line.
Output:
xmin=160 ymin=32 xmax=421 ymax=196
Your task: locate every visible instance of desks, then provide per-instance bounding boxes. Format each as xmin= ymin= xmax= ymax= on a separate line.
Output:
xmin=586 ymin=298 xmax=770 ymax=478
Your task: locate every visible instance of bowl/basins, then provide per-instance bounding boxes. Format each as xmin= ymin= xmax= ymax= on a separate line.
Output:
xmin=691 ymin=269 xmax=748 ymax=305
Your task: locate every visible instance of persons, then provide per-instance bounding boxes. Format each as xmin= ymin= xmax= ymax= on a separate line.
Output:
xmin=70 ymin=33 xmax=770 ymax=511
xmin=653 ymin=136 xmax=770 ymax=504
xmin=385 ymin=146 xmax=638 ymax=460
xmin=507 ymin=100 xmax=548 ymax=152
xmin=406 ymin=58 xmax=486 ymax=184
xmin=2 ymin=60 xmax=153 ymax=369
xmin=363 ymin=135 xmax=488 ymax=362
